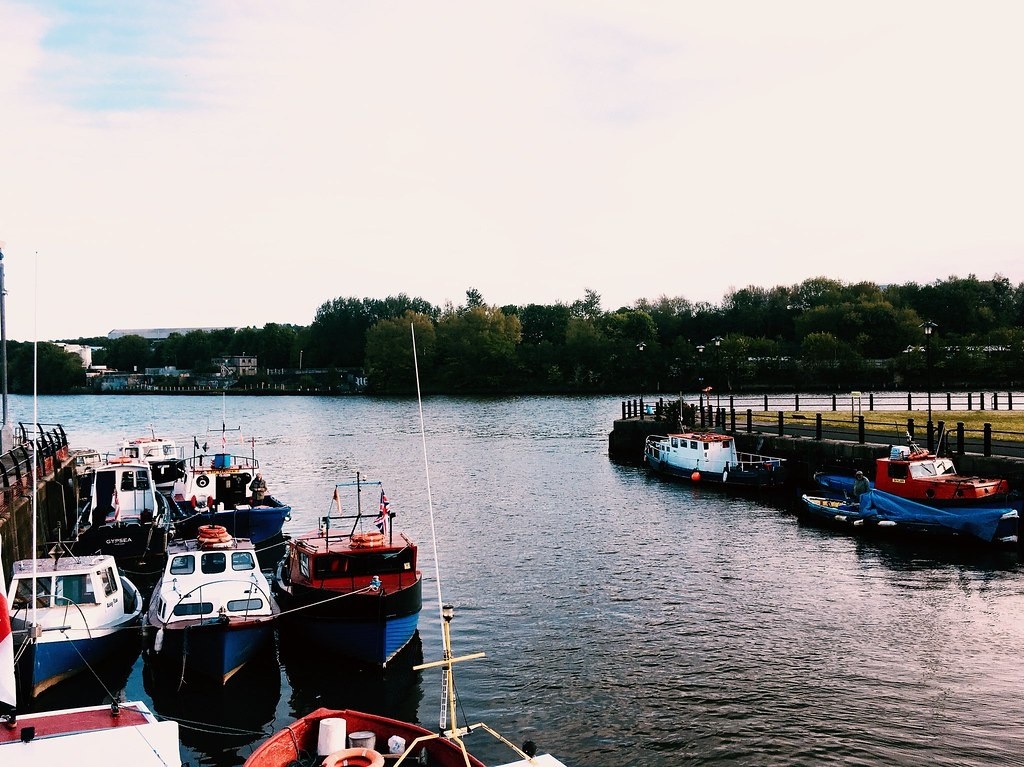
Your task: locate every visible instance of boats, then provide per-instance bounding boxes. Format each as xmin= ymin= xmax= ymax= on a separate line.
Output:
xmin=147 ymin=496 xmax=279 ymax=686
xmin=814 ymin=433 xmax=1024 ymax=515
xmin=802 ymin=493 xmax=1018 ymax=542
xmin=277 ymin=472 xmax=422 ymax=669
xmin=644 ymin=432 xmax=787 ymax=488
xmin=69 ymin=428 xmax=170 ymax=580
xmin=8 ymin=521 xmax=143 ymax=697
xmin=116 ymin=424 xmax=185 ymax=489
xmin=243 ymin=322 xmax=564 ymax=767
xmin=172 ymin=392 xmax=291 ymax=546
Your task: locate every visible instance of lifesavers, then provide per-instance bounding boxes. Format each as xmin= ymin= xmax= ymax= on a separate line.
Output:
xmin=321 ymin=743 xmax=385 ymax=767
xmin=907 ymin=448 xmax=929 ymax=459
xmin=109 ymin=456 xmax=131 ymax=464
xmin=197 ymin=525 xmax=233 ymax=548
xmin=191 ymin=490 xmax=214 ymax=512
xmin=350 ymin=530 xmax=384 ymax=548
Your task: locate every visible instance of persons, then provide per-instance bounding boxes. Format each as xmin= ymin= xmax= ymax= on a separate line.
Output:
xmin=854 ymin=471 xmax=870 ymax=503
xmin=249 ymin=473 xmax=267 ymax=507
xmin=646 ymin=404 xmax=653 ymax=414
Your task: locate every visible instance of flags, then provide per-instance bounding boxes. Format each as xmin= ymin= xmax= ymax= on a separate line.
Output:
xmin=115 ymin=498 xmax=121 ymax=521
xmin=0 ymin=561 xmax=16 ymax=707
xmin=373 ymin=491 xmax=391 ymax=535
xmin=221 ymin=432 xmax=227 ymax=453
xmin=333 ymin=488 xmax=340 ymax=511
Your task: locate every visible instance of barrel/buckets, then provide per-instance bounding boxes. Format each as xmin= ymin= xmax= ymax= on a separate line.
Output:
xmin=318 ymin=718 xmax=346 ymax=755
xmin=215 ymin=454 xmax=230 ymax=468
xmin=348 ymin=731 xmax=376 ymax=750
